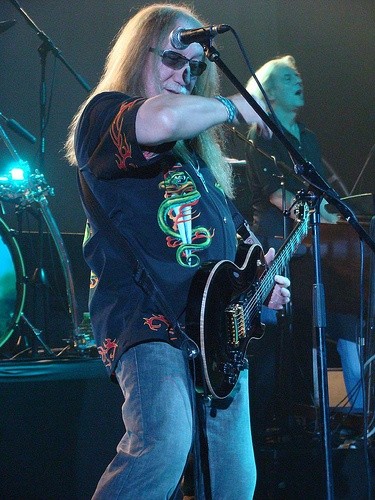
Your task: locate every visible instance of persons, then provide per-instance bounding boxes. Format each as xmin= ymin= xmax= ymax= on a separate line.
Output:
xmin=66 ymin=5 xmax=290 ymax=500
xmin=246 ymin=55 xmax=365 ymax=409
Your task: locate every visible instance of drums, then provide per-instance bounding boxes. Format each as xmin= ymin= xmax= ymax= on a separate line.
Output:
xmin=0 ymin=217 xmax=27 ymax=347
xmin=224 ymin=156 xmax=248 ymax=198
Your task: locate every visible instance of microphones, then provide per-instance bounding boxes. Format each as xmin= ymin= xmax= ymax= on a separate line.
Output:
xmin=168 ymin=24 xmax=230 ymax=50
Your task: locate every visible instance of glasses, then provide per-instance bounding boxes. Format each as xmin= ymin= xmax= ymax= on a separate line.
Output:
xmin=149 ymin=47 xmax=207 ymax=77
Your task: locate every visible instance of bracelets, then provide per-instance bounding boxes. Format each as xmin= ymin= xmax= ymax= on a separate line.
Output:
xmin=227 ymin=99 xmax=247 ymax=126
xmin=216 ymin=94 xmax=234 ymax=122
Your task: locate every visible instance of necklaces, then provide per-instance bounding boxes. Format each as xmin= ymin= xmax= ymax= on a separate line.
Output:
xmin=186 ymin=157 xmax=209 ymax=193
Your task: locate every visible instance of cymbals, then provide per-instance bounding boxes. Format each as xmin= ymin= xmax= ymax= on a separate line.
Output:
xmin=0 ymin=19 xmax=17 ymax=33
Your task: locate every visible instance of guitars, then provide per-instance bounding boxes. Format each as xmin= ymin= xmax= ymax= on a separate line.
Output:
xmin=185 ymin=191 xmax=324 ymax=402
